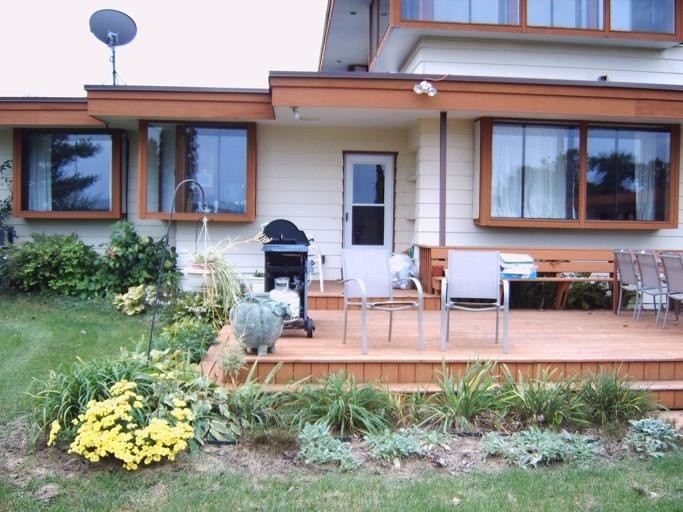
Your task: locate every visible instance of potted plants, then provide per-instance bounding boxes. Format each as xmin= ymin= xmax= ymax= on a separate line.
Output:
xmin=187 ymin=233 xmax=273 ymax=335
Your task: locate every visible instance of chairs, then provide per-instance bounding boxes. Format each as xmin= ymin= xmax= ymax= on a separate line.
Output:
xmin=615 ymin=247 xmax=683 ymax=328
xmin=337 ymin=246 xmax=426 ymax=354
xmin=439 ymin=249 xmax=510 ymax=351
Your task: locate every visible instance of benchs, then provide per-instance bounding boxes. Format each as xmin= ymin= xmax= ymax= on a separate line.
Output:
xmin=432 ymin=248 xmax=683 ymax=310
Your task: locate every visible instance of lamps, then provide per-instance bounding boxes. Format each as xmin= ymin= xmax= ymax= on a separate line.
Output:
xmin=412 ymin=80 xmax=438 ymax=97
